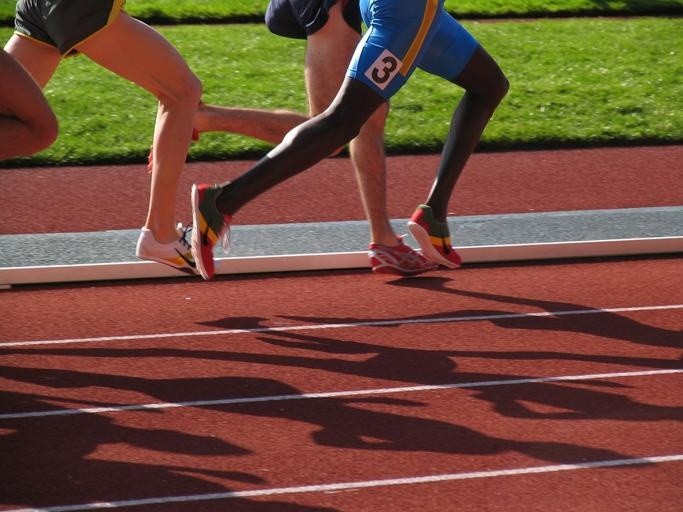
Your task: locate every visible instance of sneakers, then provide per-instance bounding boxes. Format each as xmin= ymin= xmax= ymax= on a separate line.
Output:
xmin=134 ymin=220 xmax=200 ymax=277
xmin=189 ymin=178 xmax=235 ymax=282
xmin=366 ymin=233 xmax=441 ymax=277
xmin=406 ymin=203 xmax=463 ymax=270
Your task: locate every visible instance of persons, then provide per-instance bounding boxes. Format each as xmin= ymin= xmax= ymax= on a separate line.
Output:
xmin=191 ymin=1 xmax=509 ymax=280
xmin=0 ymin=47 xmax=59 ymax=161
xmin=4 ymin=0 xmax=202 ymax=277
xmin=148 ymin=0 xmax=439 ymax=276
